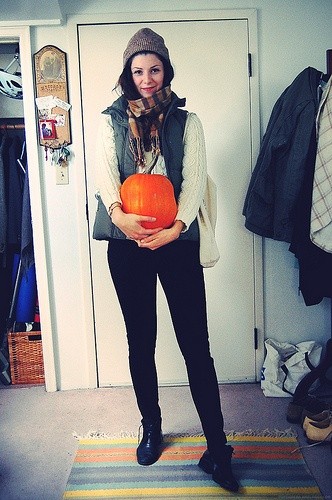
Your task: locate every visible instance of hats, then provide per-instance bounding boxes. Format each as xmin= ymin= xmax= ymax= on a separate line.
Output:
xmin=124 ymin=28 xmax=169 ymax=67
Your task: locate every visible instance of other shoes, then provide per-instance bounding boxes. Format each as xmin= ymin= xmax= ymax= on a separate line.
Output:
xmin=287 ymin=401 xmax=332 ymax=423
xmin=303 ymin=410 xmax=332 ymax=446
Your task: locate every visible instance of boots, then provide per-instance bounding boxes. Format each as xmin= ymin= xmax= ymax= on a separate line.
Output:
xmin=198 ymin=445 xmax=238 ymax=491
xmin=136 ymin=417 xmax=163 ymax=466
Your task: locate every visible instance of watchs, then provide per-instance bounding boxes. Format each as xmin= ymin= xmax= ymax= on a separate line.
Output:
xmin=109 ymin=205 xmax=121 ymax=216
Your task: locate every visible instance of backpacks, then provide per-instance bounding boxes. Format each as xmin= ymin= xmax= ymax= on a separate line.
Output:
xmin=294 ymin=338 xmax=332 ymax=398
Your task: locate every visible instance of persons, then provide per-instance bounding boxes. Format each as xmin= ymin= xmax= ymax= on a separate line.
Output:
xmin=41 ymin=123 xmax=49 ymax=137
xmin=85 ymin=27 xmax=240 ymax=492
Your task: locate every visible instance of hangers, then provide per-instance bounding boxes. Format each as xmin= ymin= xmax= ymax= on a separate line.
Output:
xmin=1 ymin=124 xmax=26 ymax=139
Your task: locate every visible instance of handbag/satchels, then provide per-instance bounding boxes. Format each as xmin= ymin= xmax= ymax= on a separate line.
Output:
xmin=260 ymin=338 xmax=322 ymax=398
xmin=197 ymin=174 xmax=220 ymax=268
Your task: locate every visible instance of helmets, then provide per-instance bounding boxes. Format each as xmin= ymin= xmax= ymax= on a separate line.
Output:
xmin=0 ymin=69 xmax=23 ymax=100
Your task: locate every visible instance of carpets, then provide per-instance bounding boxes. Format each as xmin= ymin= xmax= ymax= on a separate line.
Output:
xmin=60 ymin=430 xmax=325 ymax=500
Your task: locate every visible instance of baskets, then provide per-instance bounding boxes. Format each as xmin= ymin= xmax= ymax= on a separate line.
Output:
xmin=8 ymin=331 xmax=46 ymax=384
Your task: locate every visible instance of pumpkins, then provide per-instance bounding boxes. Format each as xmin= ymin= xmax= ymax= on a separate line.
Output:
xmin=120 ymin=172 xmax=178 ymax=231
